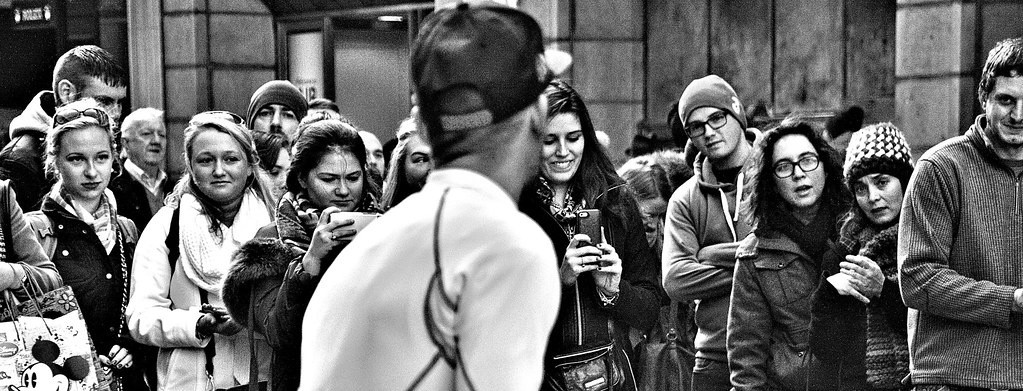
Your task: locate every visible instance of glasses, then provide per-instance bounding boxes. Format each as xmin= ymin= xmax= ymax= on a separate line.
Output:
xmin=771 ymin=155 xmax=821 ymax=178
xmin=53 ymin=108 xmax=99 ymax=128
xmin=684 ymin=111 xmax=730 ymax=138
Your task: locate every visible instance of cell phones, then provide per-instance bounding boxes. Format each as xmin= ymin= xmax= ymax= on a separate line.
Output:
xmin=329 ymin=213 xmax=381 ymax=240
xmin=577 ymin=210 xmax=603 ymax=265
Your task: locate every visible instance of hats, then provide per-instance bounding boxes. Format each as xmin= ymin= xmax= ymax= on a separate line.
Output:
xmin=678 ymin=75 xmax=747 ymax=129
xmin=410 ymin=0 xmax=554 ymax=134
xmin=247 ymin=80 xmax=308 ymax=128
xmin=843 ymin=122 xmax=915 ymax=197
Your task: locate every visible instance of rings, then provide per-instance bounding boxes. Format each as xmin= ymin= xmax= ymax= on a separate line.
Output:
xmin=582 ymin=257 xmax=585 ymax=267
xmin=330 ymin=230 xmax=337 ymax=240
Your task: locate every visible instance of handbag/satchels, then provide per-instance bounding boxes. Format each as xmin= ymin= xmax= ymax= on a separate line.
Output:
xmin=541 ymin=339 xmax=638 ymax=391
xmin=0 ymin=259 xmax=111 ymax=391
xmin=639 ymin=303 xmax=698 ymax=391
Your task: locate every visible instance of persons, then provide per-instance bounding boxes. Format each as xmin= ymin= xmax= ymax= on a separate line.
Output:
xmin=808 ymin=123 xmax=914 ymax=391
xmin=821 ymin=105 xmax=865 ymax=163
xmin=297 ymin=1 xmax=573 ymax=391
xmin=897 ymin=36 xmax=1023 ymax=391
xmin=308 ymin=100 xmax=433 ymax=214
xmin=0 ymin=45 xmax=127 ymax=214
xmin=662 ymin=75 xmax=765 ymax=391
xmin=616 ymin=100 xmax=776 ymax=391
xmin=517 ymin=78 xmax=662 ymax=391
xmin=0 ymin=178 xmax=63 ymax=313
xmin=24 ymin=99 xmax=160 ymax=391
xmin=224 ymin=120 xmax=390 ymax=391
xmin=245 ymin=81 xmax=308 ymax=204
xmin=726 ymin=122 xmax=854 ymax=391
xmin=107 ymin=107 xmax=177 ymax=234
xmin=124 ymin=111 xmax=278 ymax=391
